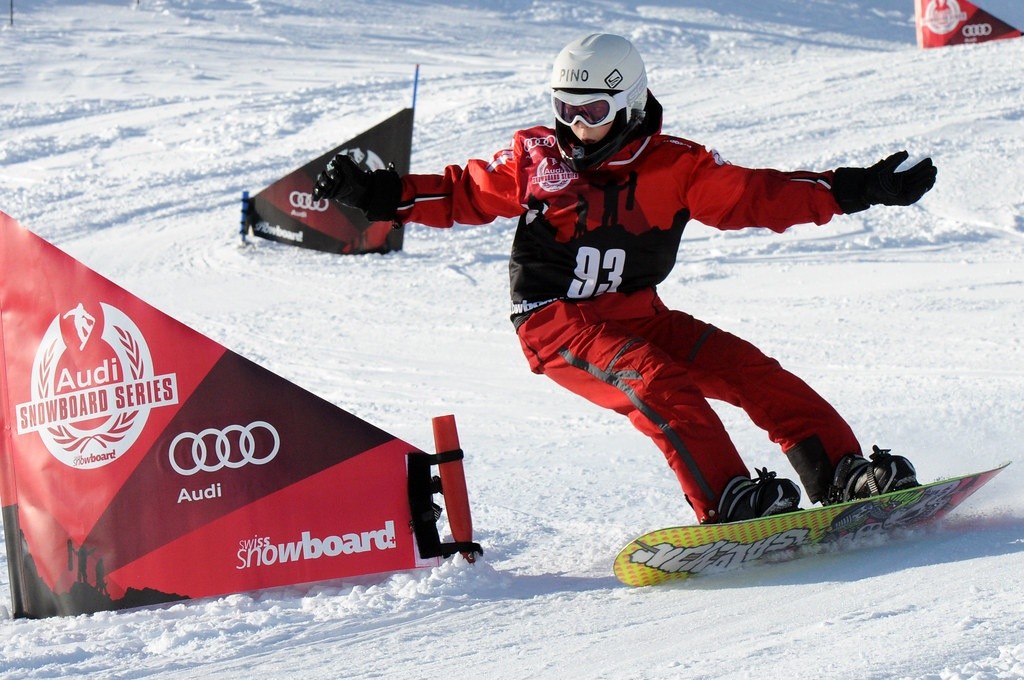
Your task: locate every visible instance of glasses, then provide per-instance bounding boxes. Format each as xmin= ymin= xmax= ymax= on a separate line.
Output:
xmin=551 ymin=90 xmax=617 ymax=128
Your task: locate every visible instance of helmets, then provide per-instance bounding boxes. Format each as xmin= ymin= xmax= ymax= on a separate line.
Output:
xmin=550 ymin=33 xmax=647 ymax=172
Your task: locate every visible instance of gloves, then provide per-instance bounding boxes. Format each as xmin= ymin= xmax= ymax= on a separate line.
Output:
xmin=831 ymin=150 xmax=937 ymax=215
xmin=312 ymin=154 xmax=402 ymax=221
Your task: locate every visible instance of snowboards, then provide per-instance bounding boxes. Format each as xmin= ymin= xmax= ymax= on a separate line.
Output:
xmin=611 ymin=458 xmax=1014 ymax=589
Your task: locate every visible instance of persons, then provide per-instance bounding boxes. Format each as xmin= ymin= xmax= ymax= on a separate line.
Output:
xmin=315 ymin=33 xmax=938 ymax=527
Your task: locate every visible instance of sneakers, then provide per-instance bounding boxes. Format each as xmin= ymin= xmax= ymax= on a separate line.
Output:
xmin=818 ymin=445 xmax=917 ymax=505
xmin=716 ymin=466 xmax=802 ymax=522
